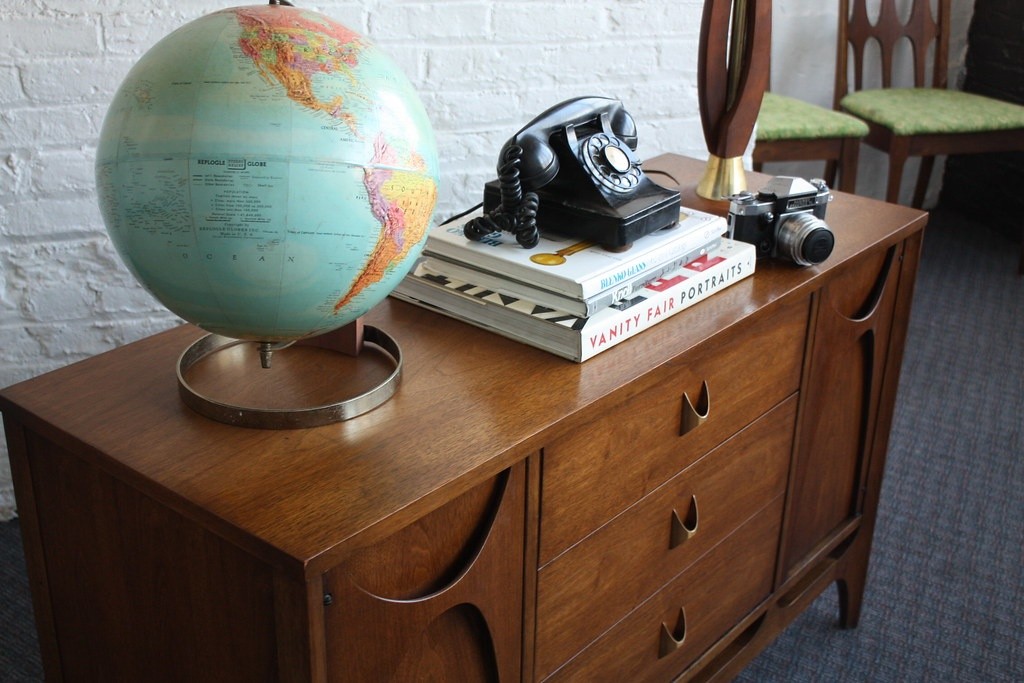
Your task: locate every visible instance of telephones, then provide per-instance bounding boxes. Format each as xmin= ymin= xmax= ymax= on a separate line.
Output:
xmin=462 ymin=93 xmax=683 ymax=253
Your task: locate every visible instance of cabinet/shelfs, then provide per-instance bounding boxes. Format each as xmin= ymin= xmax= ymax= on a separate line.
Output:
xmin=0 ymin=151 xmax=931 ymax=683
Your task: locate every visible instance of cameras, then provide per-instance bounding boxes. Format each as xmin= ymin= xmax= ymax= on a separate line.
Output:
xmin=722 ymin=175 xmax=834 ymax=268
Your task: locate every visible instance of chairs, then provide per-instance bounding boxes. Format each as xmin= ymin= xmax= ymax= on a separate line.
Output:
xmin=726 ymin=0 xmax=867 ymax=195
xmin=834 ymin=0 xmax=1023 ymax=277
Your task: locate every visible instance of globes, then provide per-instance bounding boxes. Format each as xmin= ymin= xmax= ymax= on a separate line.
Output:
xmin=93 ymin=0 xmax=441 ymax=431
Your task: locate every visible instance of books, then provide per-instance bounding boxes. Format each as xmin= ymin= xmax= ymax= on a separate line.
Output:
xmin=387 ymin=236 xmax=756 ymax=362
xmin=425 ymin=203 xmax=727 ymax=298
xmin=421 ymin=237 xmax=722 ymax=320
